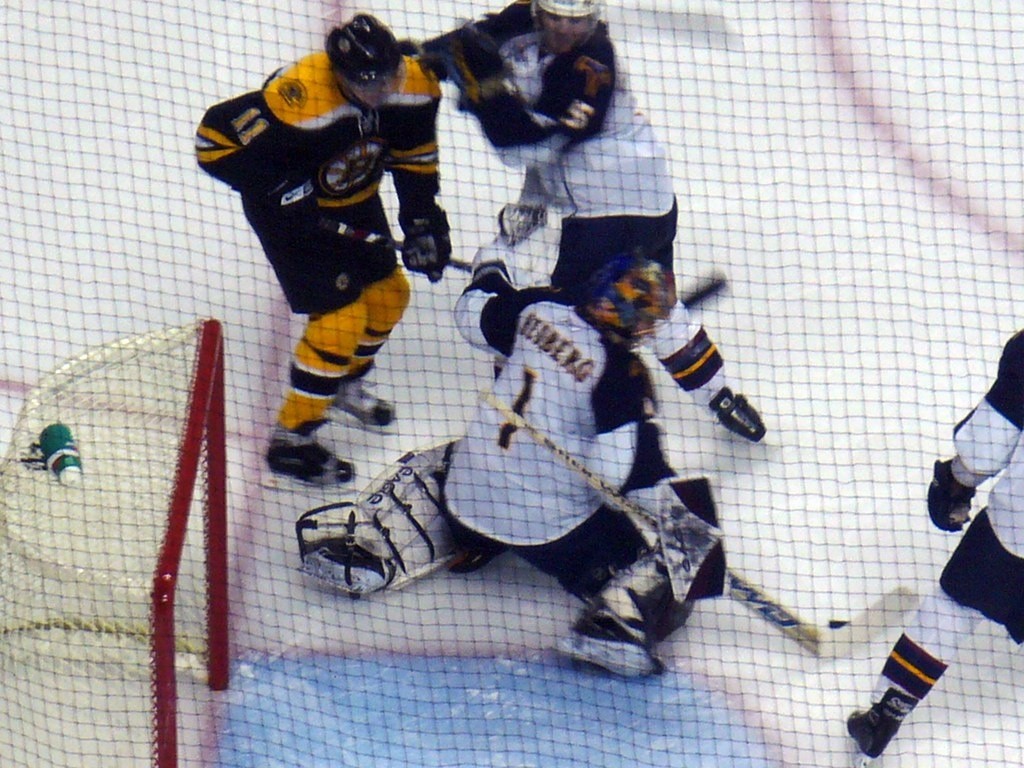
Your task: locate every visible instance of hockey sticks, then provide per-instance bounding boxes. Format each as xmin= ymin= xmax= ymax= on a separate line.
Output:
xmin=320 ymin=218 xmax=726 ymax=309
xmin=479 ymin=389 xmax=921 ymax=661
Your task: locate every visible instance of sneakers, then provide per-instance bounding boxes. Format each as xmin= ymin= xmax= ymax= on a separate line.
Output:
xmin=304 ymin=540 xmax=393 ymax=599
xmin=845 ymin=691 xmax=920 ymax=759
xmin=331 ymin=376 xmax=396 ymax=426
xmin=263 ymin=416 xmax=353 ymax=486
xmin=572 ymin=607 xmax=654 ymax=671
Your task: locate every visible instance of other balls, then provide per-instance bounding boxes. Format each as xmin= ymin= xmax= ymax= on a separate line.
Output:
xmin=829 ymin=620 xmax=849 ymax=628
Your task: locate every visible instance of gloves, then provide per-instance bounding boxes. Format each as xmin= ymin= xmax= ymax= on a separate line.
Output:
xmin=267 ymin=173 xmax=323 ymax=242
xmin=446 ymin=21 xmax=512 ymax=113
xmin=927 ymin=459 xmax=977 ymax=535
xmin=399 ymin=205 xmax=453 ymax=284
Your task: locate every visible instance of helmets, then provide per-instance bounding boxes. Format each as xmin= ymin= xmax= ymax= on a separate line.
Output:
xmin=530 ymin=0 xmax=603 ymax=56
xmin=326 ymin=13 xmax=407 ymax=108
xmin=573 ymin=254 xmax=679 ymax=351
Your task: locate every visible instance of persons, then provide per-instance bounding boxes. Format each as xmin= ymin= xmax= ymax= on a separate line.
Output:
xmin=294 ymin=200 xmax=728 ymax=676
xmin=844 ymin=327 xmax=1024 ymax=759
xmin=419 ymin=0 xmax=769 ymax=441
xmin=193 ymin=12 xmax=452 ymax=485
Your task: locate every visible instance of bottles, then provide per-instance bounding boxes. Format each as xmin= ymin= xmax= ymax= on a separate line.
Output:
xmin=40 ymin=424 xmax=81 ymax=486
